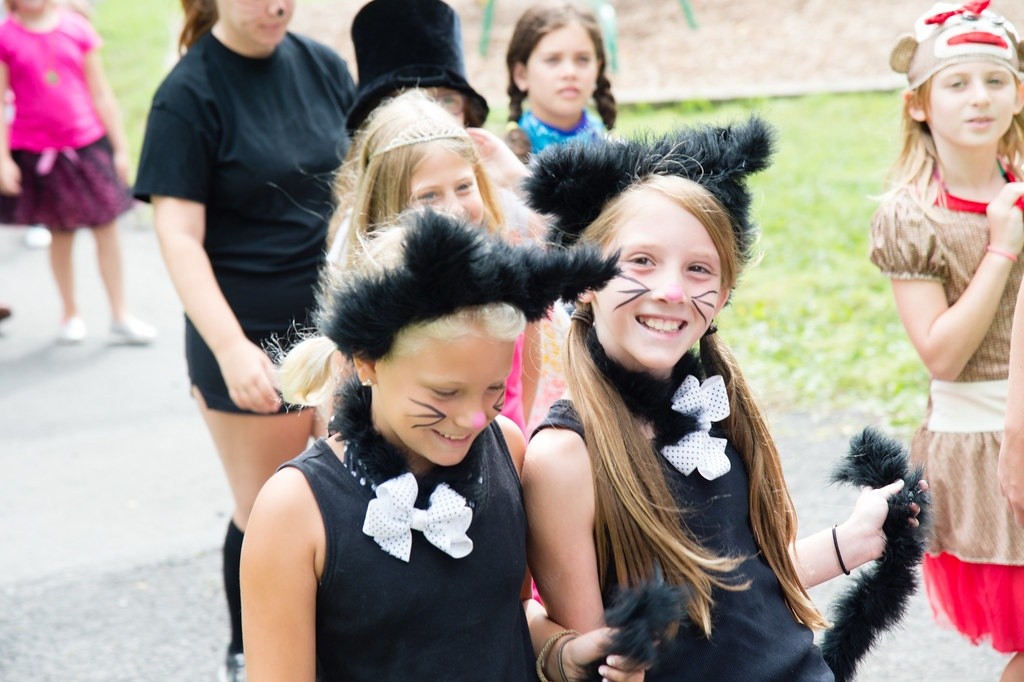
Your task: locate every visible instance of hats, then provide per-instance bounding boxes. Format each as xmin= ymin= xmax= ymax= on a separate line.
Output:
xmin=345 ymin=0 xmax=490 ymax=139
xmin=889 ymin=0 xmax=1024 ymax=88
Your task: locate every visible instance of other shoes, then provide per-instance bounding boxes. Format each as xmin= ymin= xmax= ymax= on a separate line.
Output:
xmin=112 ymin=319 xmax=155 ymax=345
xmin=61 ymin=313 xmax=82 ymax=341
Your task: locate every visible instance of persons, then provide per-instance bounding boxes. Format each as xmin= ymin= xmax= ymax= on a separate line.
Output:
xmin=998 ymin=287 xmax=1024 ymax=527
xmin=135 ymin=0 xmax=926 ymax=682
xmin=0 ymin=1 xmax=152 ymax=343
xmin=869 ymin=0 xmax=1023 ymax=682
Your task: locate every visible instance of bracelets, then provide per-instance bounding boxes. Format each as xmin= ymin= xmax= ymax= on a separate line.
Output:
xmin=536 ymin=632 xmax=582 ymax=682
xmin=832 ymin=524 xmax=853 ymax=576
xmin=558 ymin=636 xmax=578 ymax=682
xmin=987 ymin=246 xmax=1017 ymax=264
